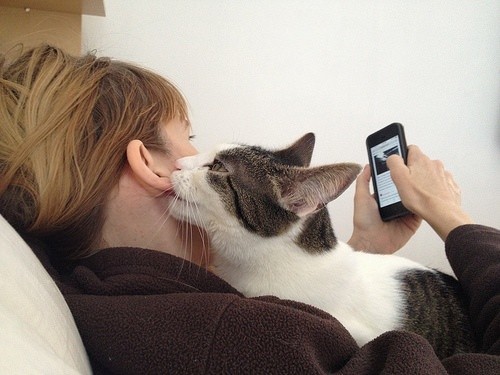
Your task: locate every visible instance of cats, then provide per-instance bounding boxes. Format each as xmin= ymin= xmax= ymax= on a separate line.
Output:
xmin=168 ymin=131 xmax=478 ymax=363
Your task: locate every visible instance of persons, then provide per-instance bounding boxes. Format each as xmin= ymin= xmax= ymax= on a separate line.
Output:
xmin=0 ymin=41 xmax=500 ymax=375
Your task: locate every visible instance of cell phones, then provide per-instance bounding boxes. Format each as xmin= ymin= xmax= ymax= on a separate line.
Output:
xmin=366 ymin=123 xmax=413 ymax=221
